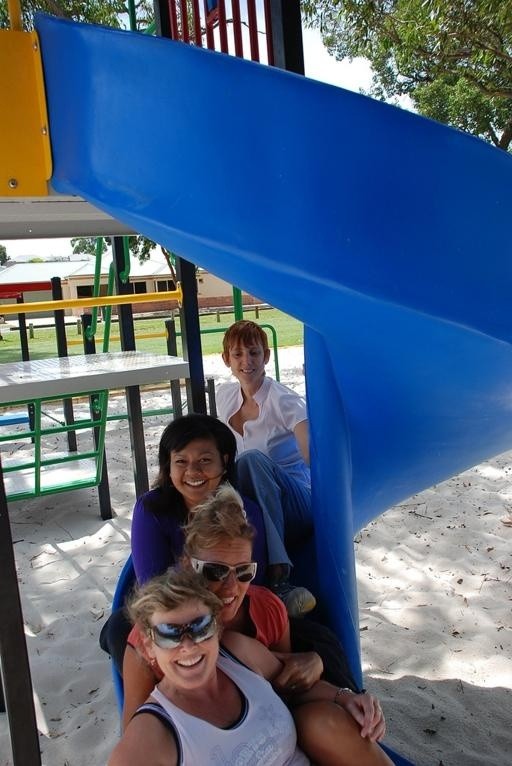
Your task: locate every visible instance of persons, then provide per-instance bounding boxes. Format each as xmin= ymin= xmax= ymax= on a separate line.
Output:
xmin=214 ymin=319 xmax=320 ymax=618
xmin=98 ymin=413 xmax=362 ymax=693
xmin=107 ymin=566 xmax=393 ymax=766
xmin=121 ymin=482 xmax=326 ymax=735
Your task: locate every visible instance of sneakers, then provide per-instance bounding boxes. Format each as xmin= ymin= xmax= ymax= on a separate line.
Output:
xmin=262 ymin=580 xmax=320 ymax=620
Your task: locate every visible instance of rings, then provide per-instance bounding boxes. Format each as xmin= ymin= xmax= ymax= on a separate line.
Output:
xmin=291 ymin=684 xmax=296 ymax=690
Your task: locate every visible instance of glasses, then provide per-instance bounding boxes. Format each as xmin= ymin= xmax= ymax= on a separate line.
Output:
xmin=188 ymin=557 xmax=258 ymax=586
xmin=144 ymin=612 xmax=217 ymax=651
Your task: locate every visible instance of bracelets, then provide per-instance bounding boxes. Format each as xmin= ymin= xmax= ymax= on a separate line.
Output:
xmin=334 ymin=686 xmax=352 ymax=703
